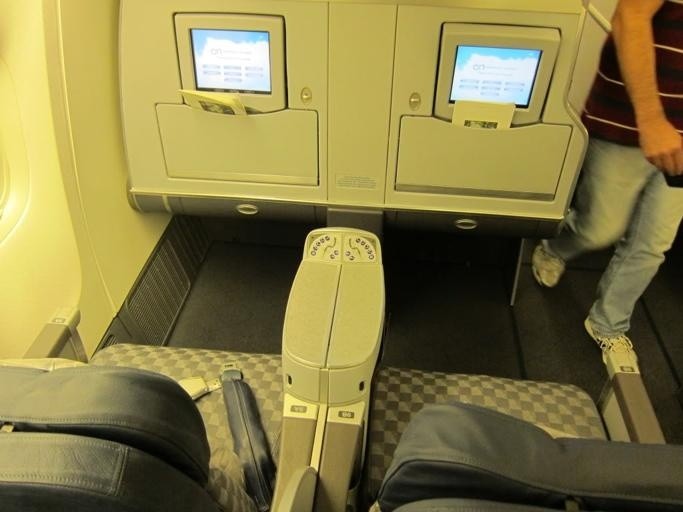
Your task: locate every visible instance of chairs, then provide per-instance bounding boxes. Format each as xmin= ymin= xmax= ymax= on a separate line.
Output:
xmin=0 ymin=227 xmax=342 ymax=512
xmin=306 ymin=229 xmax=683 ymax=512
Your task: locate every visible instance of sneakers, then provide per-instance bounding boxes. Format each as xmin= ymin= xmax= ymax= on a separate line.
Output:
xmin=584 ymin=315 xmax=638 ymax=366
xmin=531 ymin=242 xmax=566 ymax=289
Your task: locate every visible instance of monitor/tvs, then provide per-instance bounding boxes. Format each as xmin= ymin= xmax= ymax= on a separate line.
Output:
xmin=174 ymin=12 xmax=289 ymax=110
xmin=435 ymin=23 xmax=560 ymax=120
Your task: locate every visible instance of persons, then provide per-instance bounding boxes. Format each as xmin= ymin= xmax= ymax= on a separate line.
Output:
xmin=530 ymin=0 xmax=683 ymax=361
